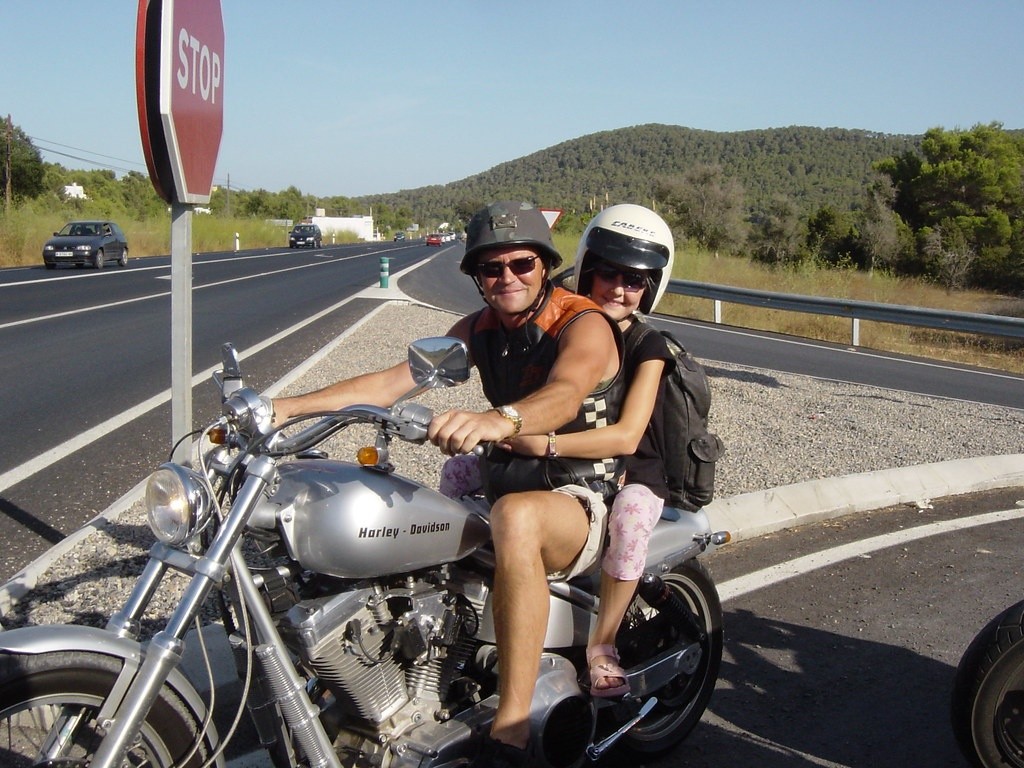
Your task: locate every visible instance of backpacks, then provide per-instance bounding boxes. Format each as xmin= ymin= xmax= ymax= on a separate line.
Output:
xmin=623 ymin=322 xmax=724 ymax=513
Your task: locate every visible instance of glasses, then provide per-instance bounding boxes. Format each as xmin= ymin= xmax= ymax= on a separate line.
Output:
xmin=473 ymin=253 xmax=540 ymax=277
xmin=593 ymin=259 xmax=648 ymax=291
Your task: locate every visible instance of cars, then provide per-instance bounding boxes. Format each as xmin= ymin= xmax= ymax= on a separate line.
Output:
xmin=394 ymin=232 xmax=405 ymax=242
xmin=419 ymin=231 xmax=456 ymax=247
xmin=42 ymin=220 xmax=129 ymax=270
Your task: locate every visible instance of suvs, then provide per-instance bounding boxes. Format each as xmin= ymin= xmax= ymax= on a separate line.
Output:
xmin=288 ymin=224 xmax=324 ymax=249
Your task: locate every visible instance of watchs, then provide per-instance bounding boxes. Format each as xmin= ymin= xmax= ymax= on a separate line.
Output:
xmin=486 ymin=404 xmax=522 ymax=443
xmin=546 ymin=431 xmax=559 ymax=460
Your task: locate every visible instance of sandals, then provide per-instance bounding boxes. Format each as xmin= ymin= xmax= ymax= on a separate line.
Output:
xmin=584 ymin=644 xmax=631 ymax=697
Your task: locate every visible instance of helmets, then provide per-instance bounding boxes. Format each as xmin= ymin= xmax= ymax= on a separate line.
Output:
xmin=459 ymin=201 xmax=563 ymax=275
xmin=574 ymin=203 xmax=675 ymax=315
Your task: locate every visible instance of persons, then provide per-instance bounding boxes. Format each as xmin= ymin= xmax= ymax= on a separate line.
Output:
xmin=257 ymin=198 xmax=625 ymax=768
xmin=489 ymin=204 xmax=677 ymax=699
xmin=294 ymin=226 xmax=301 ymax=233
xmin=75 ymin=227 xmax=84 ymax=236
xmin=95 ymin=226 xmax=112 ymax=237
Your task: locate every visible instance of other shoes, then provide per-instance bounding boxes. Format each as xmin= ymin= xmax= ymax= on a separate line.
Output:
xmin=476 ymin=730 xmax=533 ymax=768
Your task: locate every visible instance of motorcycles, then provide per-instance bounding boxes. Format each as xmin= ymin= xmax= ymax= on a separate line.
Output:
xmin=1 ymin=337 xmax=732 ymax=768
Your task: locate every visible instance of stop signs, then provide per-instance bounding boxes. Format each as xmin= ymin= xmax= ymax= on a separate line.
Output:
xmin=160 ymin=0 xmax=226 ymax=206
